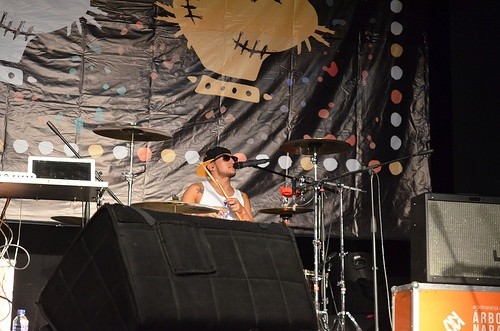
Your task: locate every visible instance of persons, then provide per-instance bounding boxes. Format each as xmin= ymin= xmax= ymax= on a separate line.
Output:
xmin=180 ymin=147 xmax=255 ymax=222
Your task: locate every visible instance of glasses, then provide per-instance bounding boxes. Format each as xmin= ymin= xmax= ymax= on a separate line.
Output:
xmin=210 ymin=155 xmax=238 ymax=164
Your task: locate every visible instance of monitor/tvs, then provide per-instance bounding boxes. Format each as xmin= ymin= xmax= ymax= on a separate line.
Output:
xmin=26 ymin=155 xmax=95 ymax=180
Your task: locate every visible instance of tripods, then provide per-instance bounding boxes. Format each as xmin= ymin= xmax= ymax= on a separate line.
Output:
xmin=320 ymin=182 xmax=365 ymax=330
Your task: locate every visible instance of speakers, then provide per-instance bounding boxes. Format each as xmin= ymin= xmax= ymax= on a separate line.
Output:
xmin=409 ymin=192 xmax=500 ymax=286
xmin=34 ymin=203 xmax=319 ymax=331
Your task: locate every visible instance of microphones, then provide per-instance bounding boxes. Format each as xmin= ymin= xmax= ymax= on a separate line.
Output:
xmin=232 ymin=159 xmax=270 ymax=169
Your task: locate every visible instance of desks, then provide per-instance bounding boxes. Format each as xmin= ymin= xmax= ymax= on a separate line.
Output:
xmin=0 ymin=177 xmax=108 ymax=233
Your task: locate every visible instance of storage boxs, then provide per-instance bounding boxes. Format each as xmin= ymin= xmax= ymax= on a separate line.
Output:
xmin=391 ymin=281 xmax=500 ymax=331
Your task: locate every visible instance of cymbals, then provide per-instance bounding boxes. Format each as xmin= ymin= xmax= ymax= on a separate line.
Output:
xmin=259 ymin=206 xmax=313 ymax=218
xmin=92 ymin=126 xmax=172 ymax=143
xmin=130 ymin=200 xmax=220 ymax=214
xmin=279 ymin=137 xmax=352 ymax=157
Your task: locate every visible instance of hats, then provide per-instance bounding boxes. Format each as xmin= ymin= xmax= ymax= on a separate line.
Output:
xmin=196 ymin=147 xmax=231 ymax=177
